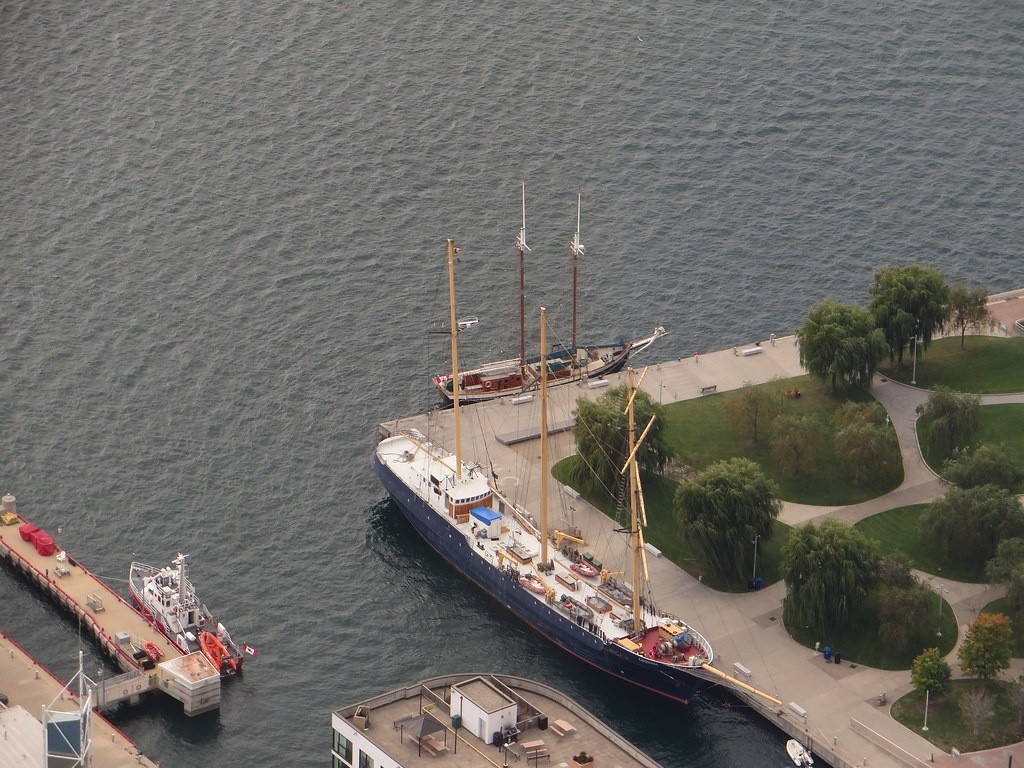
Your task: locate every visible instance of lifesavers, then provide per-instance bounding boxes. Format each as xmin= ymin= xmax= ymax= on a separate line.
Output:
xmin=485 ymin=380 xmax=493 ymax=389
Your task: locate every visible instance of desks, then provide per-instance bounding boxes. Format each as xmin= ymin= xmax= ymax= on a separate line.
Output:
xmin=416 ymin=735 xmax=431 ymax=744
xmin=426 ymin=739 xmax=446 ymax=756
xmin=522 ymin=739 xmax=545 ymax=750
xmin=554 ymin=719 xmax=573 ymax=735
xmin=504 ymin=729 xmax=521 ymax=743
xmin=56 ymin=563 xmax=69 ymax=576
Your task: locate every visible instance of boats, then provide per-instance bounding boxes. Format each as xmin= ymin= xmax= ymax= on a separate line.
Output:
xmin=127 ymin=552 xmax=248 ymax=678
xmin=786 ymin=738 xmax=814 ymax=768
xmin=200 ymin=631 xmax=238 ymax=673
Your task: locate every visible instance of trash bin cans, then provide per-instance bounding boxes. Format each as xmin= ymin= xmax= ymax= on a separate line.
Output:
xmin=493 ymin=731 xmax=503 ymax=747
xmin=836 ymin=655 xmax=841 ymax=664
xmin=452 ymin=714 xmax=461 ymax=729
xmin=538 ymin=715 xmax=548 ymax=730
xmin=824 ymin=647 xmax=832 ymax=659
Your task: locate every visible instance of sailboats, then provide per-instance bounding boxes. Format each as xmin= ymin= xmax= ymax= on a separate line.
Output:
xmin=429 ymin=188 xmax=672 ymax=404
xmin=374 ymin=236 xmax=714 ymax=706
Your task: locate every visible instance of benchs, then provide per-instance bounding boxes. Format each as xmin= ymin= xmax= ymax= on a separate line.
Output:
xmin=588 ymin=379 xmax=608 ymax=388
xmin=789 ymin=702 xmax=807 ymax=717
xmin=520 ymin=743 xmax=524 ymax=749
xmin=644 ymin=543 xmax=662 ymax=557
xmin=393 ymin=714 xmax=414 ymax=732
xmin=421 ymin=745 xmax=437 ymax=758
xmin=428 ymin=735 xmax=435 ymax=740
xmin=53 ymin=566 xmax=72 ymax=578
xmin=699 ymin=385 xmax=717 ymax=395
xmin=410 ymin=428 xmax=426 ymax=441
xmin=563 ymin=485 xmax=580 ymax=499
xmin=733 ymin=662 xmax=751 ymax=677
xmin=515 ymin=504 xmax=530 ymax=517
xmin=741 ymin=347 xmax=763 ymax=355
xmin=526 ymin=748 xmax=547 ymax=754
xmin=512 ymin=394 xmax=533 ymax=405
xmin=437 ymin=741 xmax=450 ymax=754
xmin=564 ymin=721 xmax=578 ymax=731
xmin=503 ymin=744 xmax=521 ymax=762
xmin=549 ymin=725 xmax=564 ymax=737
xmin=408 ymin=734 xmax=422 ymax=748
xmin=622 ymin=581 xmax=633 ymax=591
xmin=527 ymin=753 xmax=551 ymax=766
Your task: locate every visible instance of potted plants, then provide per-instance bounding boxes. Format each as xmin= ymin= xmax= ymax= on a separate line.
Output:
xmin=571 ymin=751 xmax=594 ymax=768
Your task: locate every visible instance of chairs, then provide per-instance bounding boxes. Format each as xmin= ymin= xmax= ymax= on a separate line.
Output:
xmin=56 ymin=551 xmax=66 ymax=563
